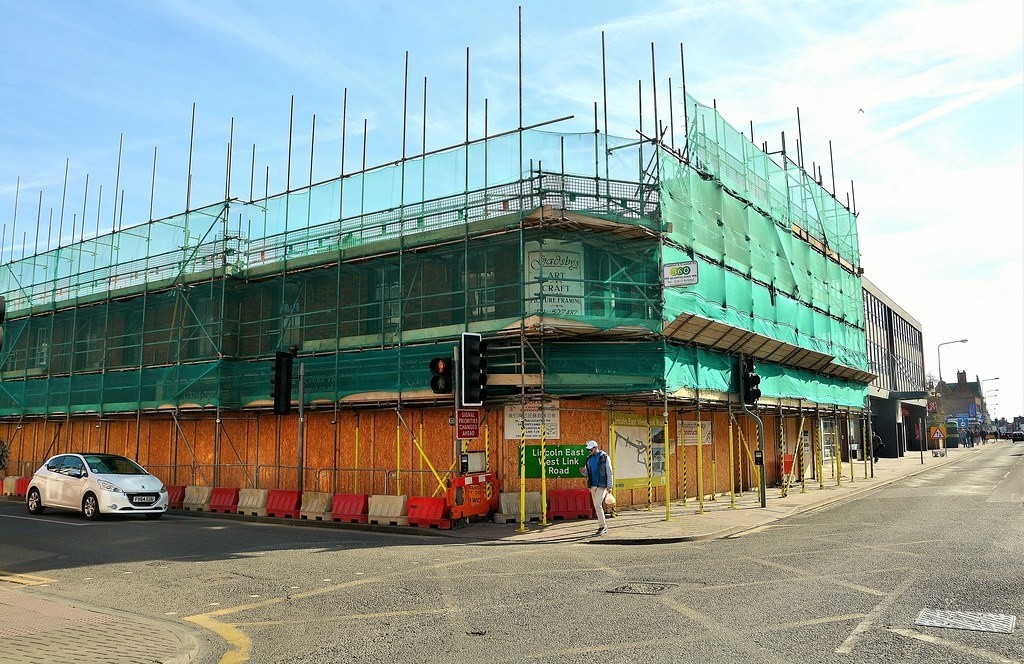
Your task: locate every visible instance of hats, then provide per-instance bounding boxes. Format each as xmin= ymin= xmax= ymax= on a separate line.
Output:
xmin=586 ymin=441 xmax=598 ymax=449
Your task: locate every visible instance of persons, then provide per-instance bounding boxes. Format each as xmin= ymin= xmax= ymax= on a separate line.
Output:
xmin=980 ymin=428 xmax=988 ymax=445
xmin=872 ymin=432 xmax=882 ymax=464
xmin=581 ymin=441 xmax=613 ymax=537
xmin=960 ymin=428 xmax=979 ymax=448
xmin=994 ymin=430 xmax=998 ymax=441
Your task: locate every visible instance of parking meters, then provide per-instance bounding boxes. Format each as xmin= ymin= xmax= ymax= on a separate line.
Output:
xmin=755 ymin=450 xmax=762 ymax=466
xmin=461 ymin=452 xmax=468 ymax=475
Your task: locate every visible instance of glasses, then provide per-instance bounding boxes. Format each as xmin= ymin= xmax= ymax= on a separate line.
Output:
xmin=587 ymin=449 xmax=592 ymax=452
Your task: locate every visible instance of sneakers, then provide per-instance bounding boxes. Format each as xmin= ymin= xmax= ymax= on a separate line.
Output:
xmin=595 ymin=527 xmax=608 ymax=535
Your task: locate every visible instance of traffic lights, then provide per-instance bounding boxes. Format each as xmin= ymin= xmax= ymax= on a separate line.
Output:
xmin=460 ymin=332 xmax=487 ymax=408
xmin=270 ymin=350 xmax=293 ymax=416
xmin=429 ymin=357 xmax=453 ymax=394
xmin=743 ymin=372 xmax=761 ymax=405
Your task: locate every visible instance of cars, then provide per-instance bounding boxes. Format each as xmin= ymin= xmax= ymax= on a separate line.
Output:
xmin=25 ymin=452 xmax=169 ymax=521
xmin=1000 ymin=431 xmax=1024 ymax=443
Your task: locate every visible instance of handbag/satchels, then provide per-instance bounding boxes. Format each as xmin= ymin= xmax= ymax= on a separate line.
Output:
xmin=605 ymin=491 xmax=616 ymax=505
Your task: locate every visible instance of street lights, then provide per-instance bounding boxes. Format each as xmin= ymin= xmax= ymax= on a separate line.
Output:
xmin=981 ymin=377 xmax=999 ymax=410
xmin=938 ymin=339 xmax=968 ymax=382
